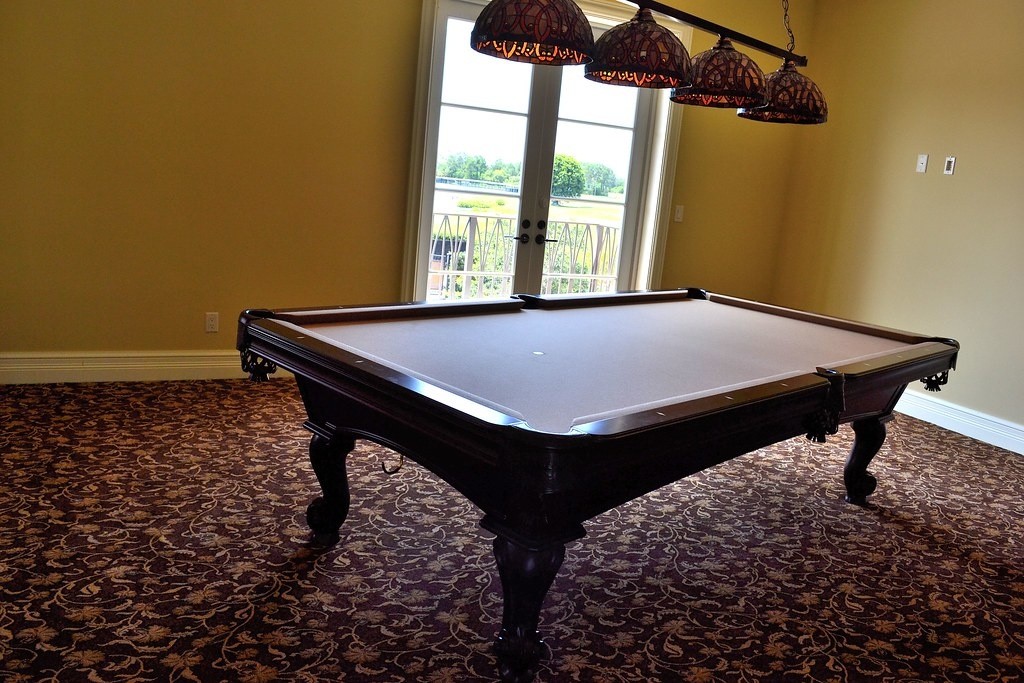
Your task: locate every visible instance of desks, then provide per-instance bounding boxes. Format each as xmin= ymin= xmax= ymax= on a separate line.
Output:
xmin=236 ymin=287 xmax=961 ymax=683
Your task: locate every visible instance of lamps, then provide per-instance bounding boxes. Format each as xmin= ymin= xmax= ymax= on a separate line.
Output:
xmin=583 ymin=7 xmax=695 ymax=89
xmin=471 ymin=0 xmax=596 ymax=66
xmin=669 ymin=35 xmax=768 ymax=109
xmin=737 ymin=60 xmax=828 ymax=125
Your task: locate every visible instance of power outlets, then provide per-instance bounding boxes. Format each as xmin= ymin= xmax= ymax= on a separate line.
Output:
xmin=206 ymin=312 xmax=218 ymax=333
